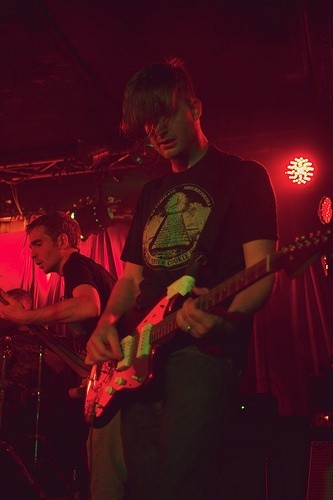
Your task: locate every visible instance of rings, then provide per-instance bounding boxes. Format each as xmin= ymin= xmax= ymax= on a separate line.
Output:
xmin=184 ymin=324 xmax=191 ymax=335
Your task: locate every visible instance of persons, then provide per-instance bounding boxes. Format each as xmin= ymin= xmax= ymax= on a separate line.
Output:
xmin=84 ymin=58 xmax=276 ymax=500
xmin=0 ymin=211 xmax=130 ymax=500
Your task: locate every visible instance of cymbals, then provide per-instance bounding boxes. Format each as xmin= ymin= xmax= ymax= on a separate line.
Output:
xmin=0 ymin=334 xmax=80 ymax=362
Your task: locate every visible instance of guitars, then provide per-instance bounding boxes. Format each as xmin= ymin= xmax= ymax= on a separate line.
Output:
xmin=84 ymin=229 xmax=333 ymax=429
xmin=0 ymin=287 xmax=91 ymax=377
